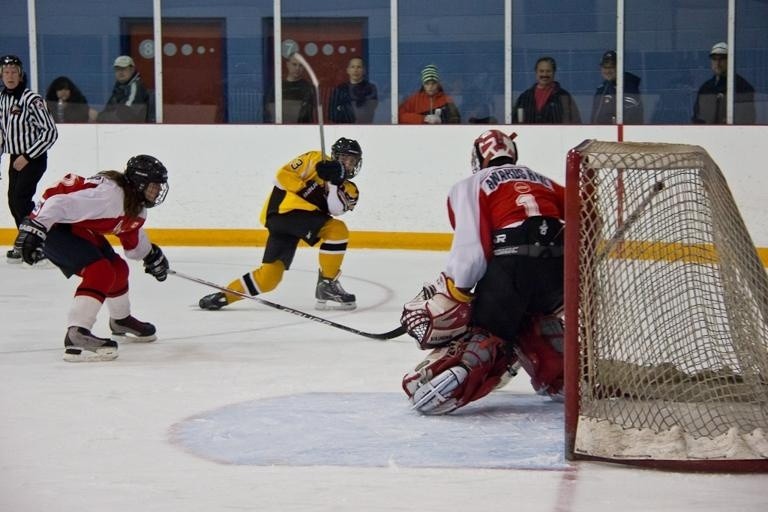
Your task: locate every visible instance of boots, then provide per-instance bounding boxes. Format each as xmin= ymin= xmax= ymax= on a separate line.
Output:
xmin=315 ymin=268 xmax=355 ymax=302
xmin=6 ymin=248 xmax=21 ymax=259
xmin=199 ymin=292 xmax=227 ymax=309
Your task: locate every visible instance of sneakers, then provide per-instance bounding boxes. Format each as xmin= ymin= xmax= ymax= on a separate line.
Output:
xmin=109 ymin=315 xmax=154 ymax=337
xmin=64 ymin=326 xmax=117 ymax=349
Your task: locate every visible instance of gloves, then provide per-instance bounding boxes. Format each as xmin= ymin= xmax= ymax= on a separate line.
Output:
xmin=142 ymin=244 xmax=168 ymax=281
xmin=423 ymin=115 xmax=441 ymax=124
xmin=315 ymin=159 xmax=345 ymax=186
xmin=295 ymin=179 xmax=328 ymax=212
xmin=13 ymin=218 xmax=47 ymax=265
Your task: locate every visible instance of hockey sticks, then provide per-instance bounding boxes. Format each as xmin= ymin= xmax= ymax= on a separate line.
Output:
xmin=293 ymin=51 xmax=326 ymax=160
xmin=170 ymin=269 xmax=407 ymax=340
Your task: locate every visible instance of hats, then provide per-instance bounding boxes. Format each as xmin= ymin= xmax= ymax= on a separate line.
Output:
xmin=420 ymin=64 xmax=439 ymax=83
xmin=599 ymin=50 xmax=615 ymax=66
xmin=113 ymin=55 xmax=135 ymax=68
xmin=709 ymin=41 xmax=727 ymax=56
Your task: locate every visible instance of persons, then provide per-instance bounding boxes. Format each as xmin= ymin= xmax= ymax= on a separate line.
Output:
xmin=401 ymin=131 xmax=591 ymax=416
xmin=13 ymin=155 xmax=169 ymax=362
xmin=398 ymin=64 xmax=460 ymax=124
xmin=198 ymin=137 xmax=363 ymax=312
xmin=96 ymin=55 xmax=150 ymax=122
xmin=692 ymin=41 xmax=756 ymax=125
xmin=45 ymin=76 xmax=97 ymax=123
xmin=262 ymin=55 xmax=314 ymax=124
xmin=328 ymin=55 xmax=378 ymax=124
xmin=512 ymin=57 xmax=581 ymax=124
xmin=0 ymin=55 xmax=59 ymax=264
xmin=591 ymin=51 xmax=642 ymax=124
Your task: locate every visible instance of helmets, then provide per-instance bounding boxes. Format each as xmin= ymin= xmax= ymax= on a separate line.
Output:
xmin=470 ymin=129 xmax=516 ymax=174
xmin=0 ymin=55 xmax=23 ymax=77
xmin=330 ymin=137 xmax=362 ymax=178
xmin=125 ymin=154 xmax=168 ymax=204
